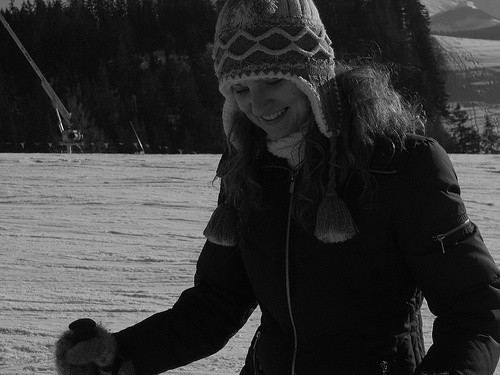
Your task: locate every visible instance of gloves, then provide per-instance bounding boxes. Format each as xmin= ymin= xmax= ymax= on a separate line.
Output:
xmin=55 ymin=318 xmax=135 ymax=375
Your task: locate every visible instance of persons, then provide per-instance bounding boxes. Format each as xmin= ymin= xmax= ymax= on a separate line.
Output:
xmin=55 ymin=0 xmax=500 ymax=375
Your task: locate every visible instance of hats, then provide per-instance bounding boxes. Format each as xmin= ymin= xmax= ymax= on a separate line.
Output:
xmin=203 ymin=0 xmax=360 ymax=247
